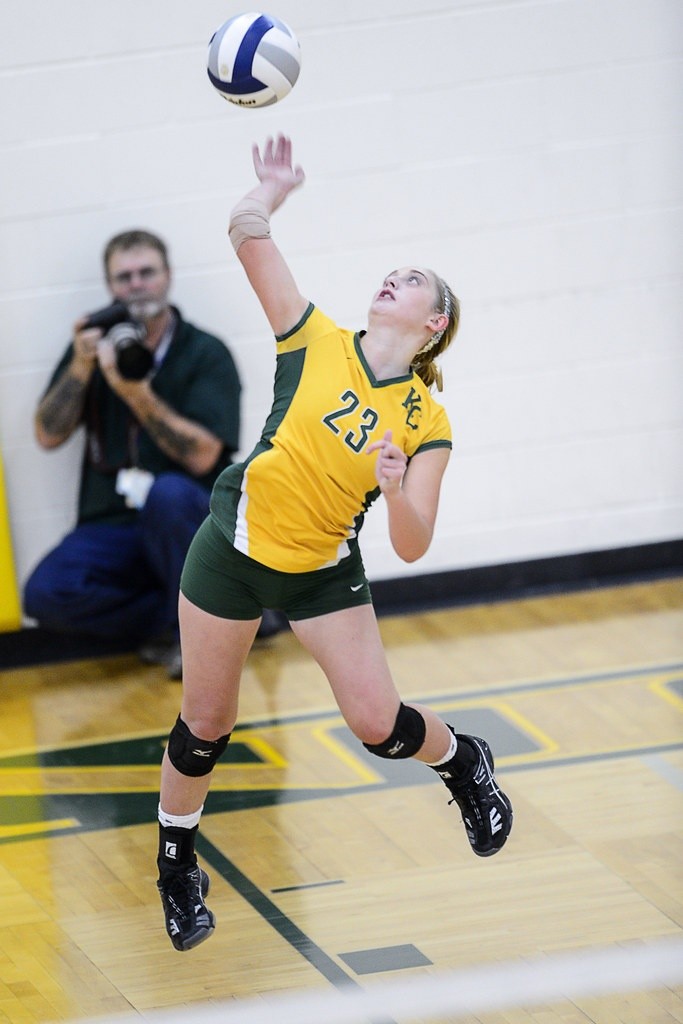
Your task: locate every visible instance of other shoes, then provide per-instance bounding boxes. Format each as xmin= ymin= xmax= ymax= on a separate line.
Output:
xmin=144 ymin=645 xmax=183 ymax=683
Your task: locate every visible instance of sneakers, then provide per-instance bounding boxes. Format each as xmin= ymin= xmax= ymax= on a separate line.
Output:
xmin=157 ymin=853 xmax=216 ymax=951
xmin=450 ymin=734 xmax=513 ymax=857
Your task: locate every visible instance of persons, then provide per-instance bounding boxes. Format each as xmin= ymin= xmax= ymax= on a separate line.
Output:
xmin=156 ymin=132 xmax=515 ymax=951
xmin=19 ymin=229 xmax=284 ymax=681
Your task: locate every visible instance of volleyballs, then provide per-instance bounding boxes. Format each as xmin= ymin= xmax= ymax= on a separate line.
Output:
xmin=206 ymin=10 xmax=302 ymax=110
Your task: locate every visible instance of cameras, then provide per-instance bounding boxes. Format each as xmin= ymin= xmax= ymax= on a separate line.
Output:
xmin=81 ymin=300 xmax=154 ymax=382
